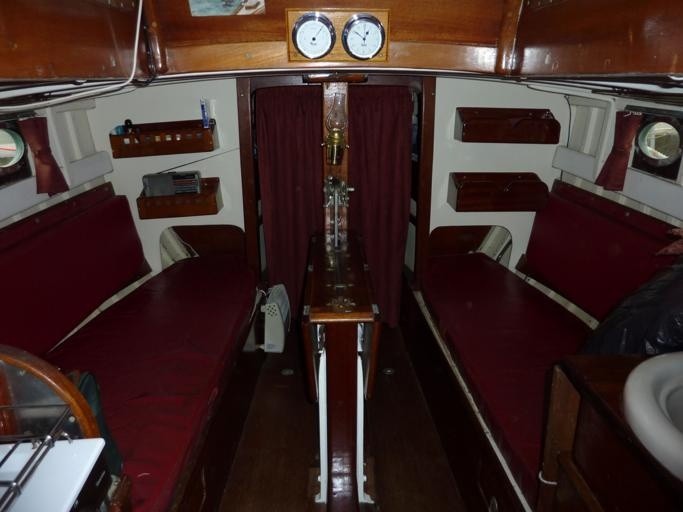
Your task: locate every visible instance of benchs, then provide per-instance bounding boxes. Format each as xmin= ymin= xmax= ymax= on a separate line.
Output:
xmin=0 ymin=182 xmax=267 ymax=512
xmin=410 ymin=178 xmax=683 ymax=512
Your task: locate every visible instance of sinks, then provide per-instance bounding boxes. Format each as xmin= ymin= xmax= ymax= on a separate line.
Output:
xmin=625 ymin=350 xmax=683 ymax=483
